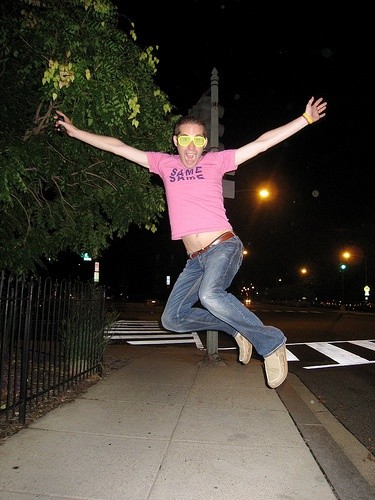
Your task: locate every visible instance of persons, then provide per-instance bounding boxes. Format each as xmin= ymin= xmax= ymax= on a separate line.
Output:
xmin=54 ymin=97 xmax=327 ymax=388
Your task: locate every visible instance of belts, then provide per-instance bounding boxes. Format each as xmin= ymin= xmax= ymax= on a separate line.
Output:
xmin=187 ymin=231 xmax=237 ymax=259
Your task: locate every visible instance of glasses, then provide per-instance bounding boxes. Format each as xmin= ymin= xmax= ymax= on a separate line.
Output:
xmin=177 ymin=135 xmax=206 ymax=148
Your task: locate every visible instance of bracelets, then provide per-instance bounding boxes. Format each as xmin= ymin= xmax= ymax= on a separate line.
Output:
xmin=302 ymin=113 xmax=313 ymax=124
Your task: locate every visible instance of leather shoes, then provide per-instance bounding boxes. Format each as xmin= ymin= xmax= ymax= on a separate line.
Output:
xmin=234 ymin=332 xmax=253 ymax=365
xmin=264 ymin=342 xmax=289 ymax=388
